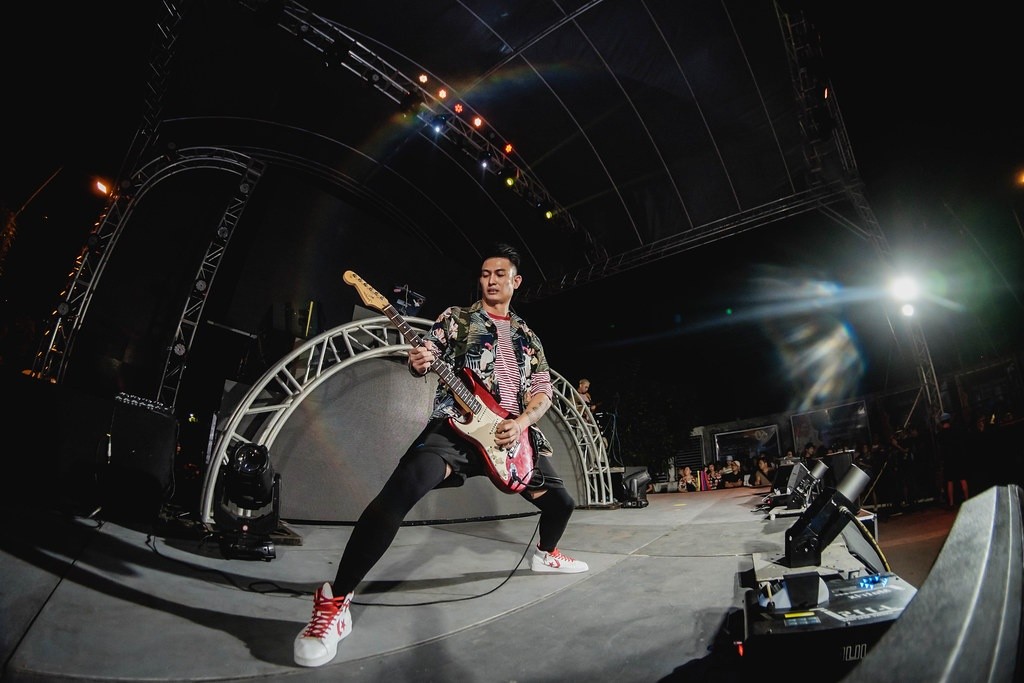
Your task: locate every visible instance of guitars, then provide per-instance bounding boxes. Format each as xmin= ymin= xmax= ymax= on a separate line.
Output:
xmin=342 ymin=270 xmax=554 ymax=495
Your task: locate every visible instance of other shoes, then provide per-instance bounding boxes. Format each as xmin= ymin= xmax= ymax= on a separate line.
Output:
xmin=944 ymin=504 xmax=954 ymax=509
xmin=891 ymin=512 xmax=903 ymax=516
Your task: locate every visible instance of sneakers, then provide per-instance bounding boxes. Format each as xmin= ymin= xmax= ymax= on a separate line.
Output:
xmin=531 ymin=543 xmax=588 ymax=573
xmin=294 ymin=582 xmax=355 ymax=667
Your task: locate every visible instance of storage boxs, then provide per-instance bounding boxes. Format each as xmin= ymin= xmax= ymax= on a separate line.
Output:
xmin=855 ymin=509 xmax=878 ymax=544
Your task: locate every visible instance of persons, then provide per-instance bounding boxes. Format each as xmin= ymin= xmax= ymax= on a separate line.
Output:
xmin=827 ymin=413 xmax=1024 ymax=515
xmin=293 ymin=244 xmax=588 ymax=667
xmin=568 ymin=379 xmax=596 ymax=433
xmin=642 ymin=441 xmax=824 ymax=494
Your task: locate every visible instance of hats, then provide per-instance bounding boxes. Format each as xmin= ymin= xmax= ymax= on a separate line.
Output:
xmin=731 ymin=461 xmax=740 ymax=467
xmin=727 ymin=456 xmax=733 ymax=460
xmin=896 ymin=425 xmax=904 ymax=433
xmin=941 ymin=413 xmax=951 ymax=421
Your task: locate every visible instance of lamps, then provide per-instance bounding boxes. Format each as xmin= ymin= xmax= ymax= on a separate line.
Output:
xmin=786 ymin=459 xmax=829 ymax=509
xmin=785 ymin=463 xmax=891 ymax=572
xmin=400 ymin=91 xmax=423 ymax=123
xmin=621 ymin=470 xmax=652 ymax=508
xmin=215 ymin=440 xmax=282 ymax=562
